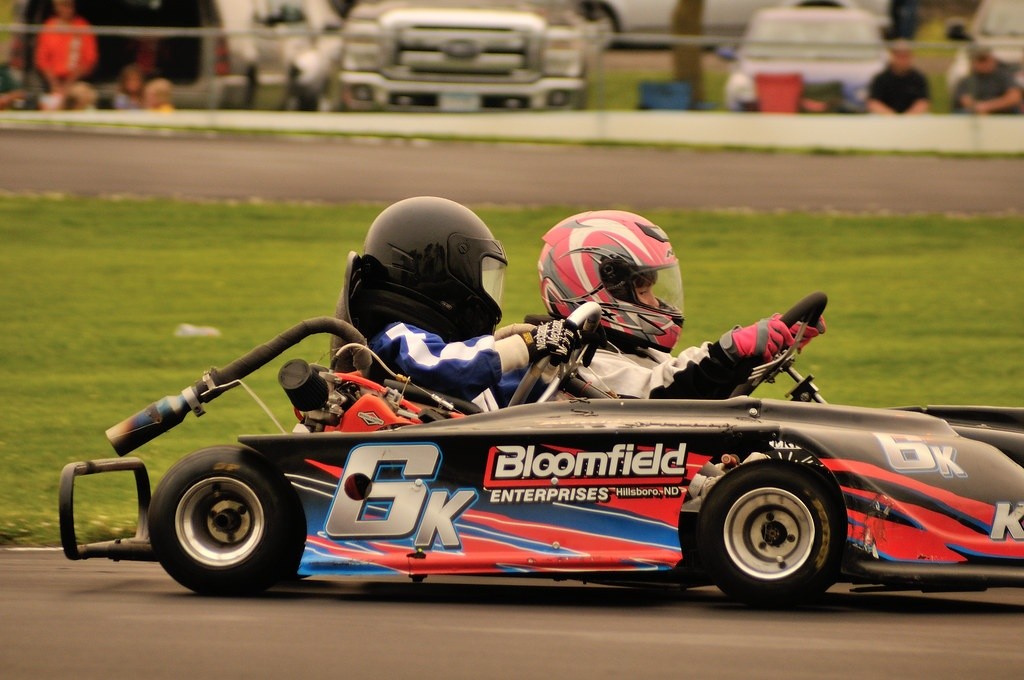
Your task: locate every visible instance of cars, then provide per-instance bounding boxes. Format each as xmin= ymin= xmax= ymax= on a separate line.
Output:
xmin=716 ymin=8 xmax=899 ymax=114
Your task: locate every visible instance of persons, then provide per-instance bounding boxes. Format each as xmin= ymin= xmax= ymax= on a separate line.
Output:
xmin=351 ymin=195 xmax=599 ymax=416
xmin=538 ymin=210 xmax=826 ymax=400
xmin=1 ymin=38 xmax=51 ymax=110
xmin=864 ymin=41 xmax=930 ymax=115
xmin=948 ymin=46 xmax=1023 ymax=113
xmin=110 ymin=65 xmax=176 ymax=110
xmin=35 ymin=1 xmax=97 ymax=90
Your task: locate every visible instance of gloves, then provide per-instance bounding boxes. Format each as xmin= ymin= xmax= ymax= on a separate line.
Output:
xmin=719 ymin=312 xmax=794 ymax=362
xmin=770 ymin=313 xmax=825 ymax=353
xmin=532 ymin=318 xmax=582 ymax=366
xmin=580 ymin=322 xmax=607 ymax=368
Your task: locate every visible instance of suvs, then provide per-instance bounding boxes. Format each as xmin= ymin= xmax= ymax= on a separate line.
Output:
xmin=341 ymin=0 xmax=586 ymax=113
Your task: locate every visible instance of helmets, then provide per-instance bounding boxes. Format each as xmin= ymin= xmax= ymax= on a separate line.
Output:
xmin=538 ymin=208 xmax=685 ymax=355
xmin=351 ymin=195 xmax=507 ymax=345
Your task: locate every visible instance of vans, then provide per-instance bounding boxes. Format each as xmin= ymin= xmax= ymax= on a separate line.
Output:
xmin=7 ymin=1 xmax=290 ymax=108
